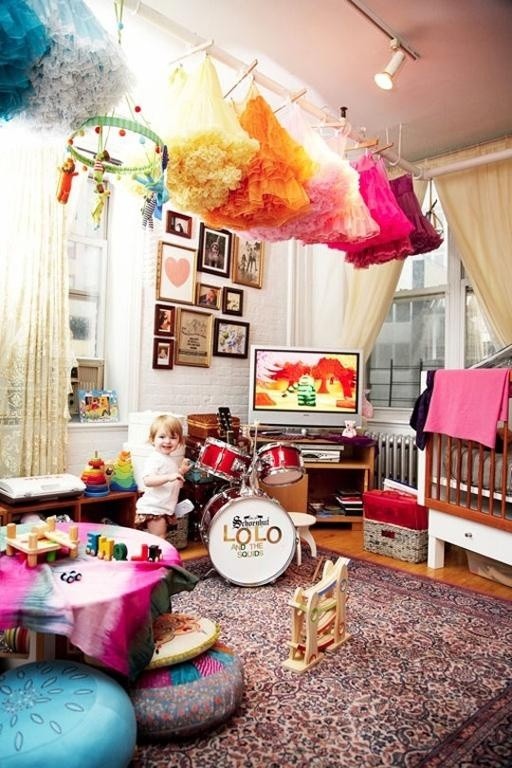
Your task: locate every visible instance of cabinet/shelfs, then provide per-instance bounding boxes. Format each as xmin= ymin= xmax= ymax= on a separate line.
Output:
xmin=241 ymin=433 xmax=375 ymax=533
xmin=0 ymin=490 xmax=144 ymax=529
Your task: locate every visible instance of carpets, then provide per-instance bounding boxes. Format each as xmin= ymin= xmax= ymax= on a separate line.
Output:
xmin=126 ymin=536 xmax=512 ymax=767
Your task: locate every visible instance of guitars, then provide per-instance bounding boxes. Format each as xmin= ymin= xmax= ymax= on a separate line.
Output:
xmin=208 ymin=406 xmax=256 ymax=502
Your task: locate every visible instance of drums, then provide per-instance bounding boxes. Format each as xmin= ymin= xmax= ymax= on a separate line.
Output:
xmin=192 ymin=435 xmax=251 ymax=485
xmin=199 ymin=486 xmax=297 ymax=589
xmin=251 ymin=440 xmax=306 ymax=488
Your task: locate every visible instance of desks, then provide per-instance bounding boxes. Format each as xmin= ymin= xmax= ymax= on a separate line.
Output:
xmin=0 ymin=522 xmax=181 ymax=659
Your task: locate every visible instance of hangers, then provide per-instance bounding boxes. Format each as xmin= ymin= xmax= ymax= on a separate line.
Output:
xmin=346 ymin=136 xmax=379 ymax=151
xmin=222 ymin=59 xmax=259 ymax=101
xmin=365 ymin=142 xmax=394 ymax=158
xmin=162 ymin=35 xmax=215 ymax=62
xmin=305 ymin=105 xmax=347 ymax=128
xmin=390 ymin=165 xmax=413 ymax=181
xmin=273 ymin=88 xmax=308 ymax=113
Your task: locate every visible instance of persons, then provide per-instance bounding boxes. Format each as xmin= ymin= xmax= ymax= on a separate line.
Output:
xmin=134 ymin=415 xmax=194 ymax=540
xmin=90 ymin=399 xmax=98 ymax=411
xmin=158 ymin=348 xmax=167 ymax=359
xmin=199 ymin=290 xmax=217 ymax=305
xmin=238 ymin=254 xmax=247 ymax=272
xmin=159 ymin=311 xmax=170 ymax=330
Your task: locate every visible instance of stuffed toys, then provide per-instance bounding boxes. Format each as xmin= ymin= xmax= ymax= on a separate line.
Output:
xmin=131 ymin=168 xmax=172 ymax=233
xmin=56 ymin=158 xmax=79 ymax=204
xmin=91 ymin=184 xmax=110 ymax=231
xmin=93 ymin=150 xmax=110 ymax=182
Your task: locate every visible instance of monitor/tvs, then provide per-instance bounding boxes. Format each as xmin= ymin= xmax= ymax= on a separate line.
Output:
xmin=245 ymin=343 xmax=364 ymax=434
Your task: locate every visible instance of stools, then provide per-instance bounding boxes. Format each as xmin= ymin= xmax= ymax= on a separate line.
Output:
xmin=286 ymin=512 xmax=317 ymax=567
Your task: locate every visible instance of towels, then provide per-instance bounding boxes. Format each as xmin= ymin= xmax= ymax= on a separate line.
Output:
xmin=423 ymin=368 xmax=510 ymax=450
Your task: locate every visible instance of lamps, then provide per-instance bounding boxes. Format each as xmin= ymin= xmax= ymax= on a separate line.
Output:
xmin=373 ymin=50 xmax=408 ymax=91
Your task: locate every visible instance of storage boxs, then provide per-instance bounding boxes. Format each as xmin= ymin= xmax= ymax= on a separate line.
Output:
xmin=362 ymin=491 xmax=428 ymax=564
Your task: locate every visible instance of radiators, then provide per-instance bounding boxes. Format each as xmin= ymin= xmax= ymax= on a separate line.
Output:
xmin=361 ymin=424 xmax=424 ymax=507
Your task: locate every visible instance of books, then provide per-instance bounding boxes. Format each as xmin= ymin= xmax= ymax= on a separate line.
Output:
xmin=309 ymin=487 xmax=364 ymax=518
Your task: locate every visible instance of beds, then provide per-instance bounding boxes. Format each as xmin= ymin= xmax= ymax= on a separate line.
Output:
xmin=424 ymin=371 xmax=512 ymax=569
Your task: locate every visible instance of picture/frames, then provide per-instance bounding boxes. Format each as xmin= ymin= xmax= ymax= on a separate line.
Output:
xmin=196 ymin=281 xmax=222 ymax=311
xmin=214 ymin=318 xmax=250 ymax=359
xmin=152 ymin=338 xmax=175 ymax=369
xmin=175 ymin=307 xmax=214 ymax=369
xmin=77 ymin=389 xmax=118 ymax=422
xmin=196 ymin=222 xmax=232 ymax=277
xmin=232 ymin=230 xmax=264 ymax=290
xmin=154 ymin=304 xmax=175 ymax=337
xmin=156 ymin=239 xmax=198 ymax=307
xmin=221 ymin=287 xmax=244 ymax=315
xmin=166 ymin=210 xmax=192 ymax=239
